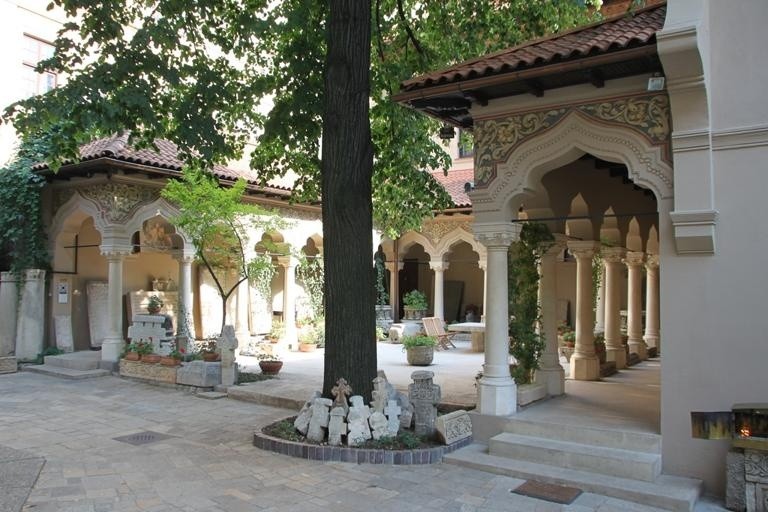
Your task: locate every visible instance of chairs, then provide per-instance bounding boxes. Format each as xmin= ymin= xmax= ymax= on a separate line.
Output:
xmin=421 ymin=317 xmax=458 ymax=353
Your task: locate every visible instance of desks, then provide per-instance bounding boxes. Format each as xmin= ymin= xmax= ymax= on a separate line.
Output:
xmin=448 ymin=322 xmax=487 ymax=352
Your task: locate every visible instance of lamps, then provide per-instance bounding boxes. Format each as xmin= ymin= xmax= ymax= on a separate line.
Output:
xmin=440 ymin=121 xmax=454 ymax=139
xmin=647 ymin=72 xmax=665 ymax=91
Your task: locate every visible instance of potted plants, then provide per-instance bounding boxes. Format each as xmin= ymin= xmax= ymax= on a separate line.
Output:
xmin=121 ymin=336 xmax=219 ymax=366
xmin=256 ymin=257 xmax=439 ymax=375
xmin=563 ymin=330 xmax=629 ymax=354
xmin=146 ymin=295 xmax=164 ymax=314
